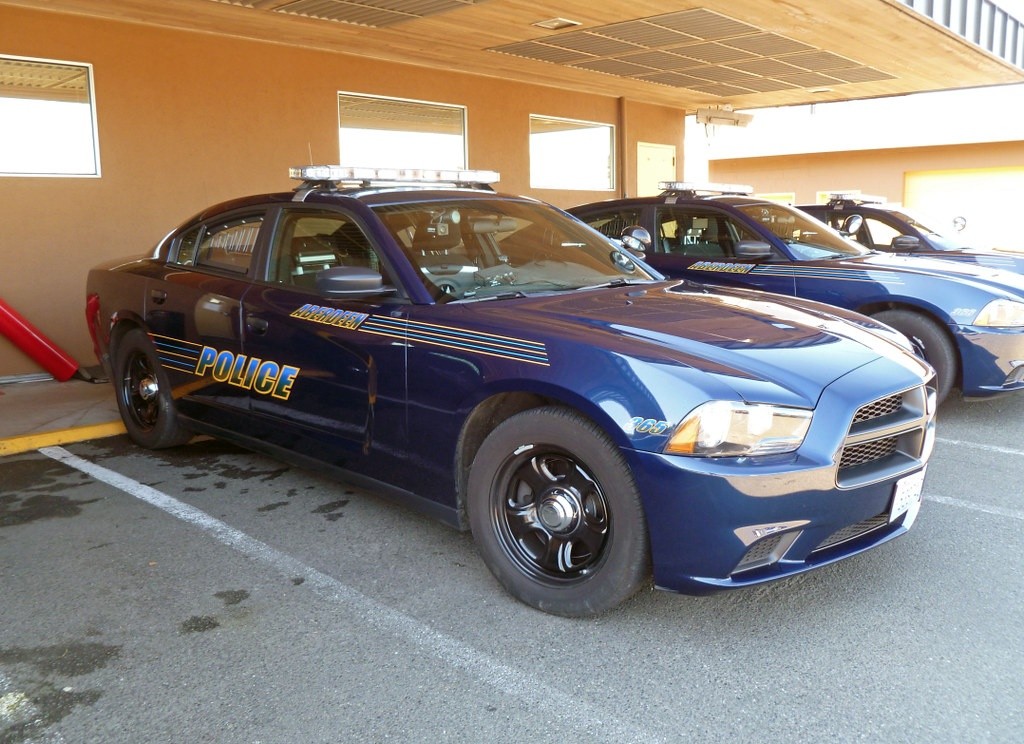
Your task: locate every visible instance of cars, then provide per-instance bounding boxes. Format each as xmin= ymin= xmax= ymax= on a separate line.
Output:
xmin=788 ymin=190 xmax=1024 ymax=289
xmin=86 ymin=161 xmax=937 ymax=621
xmin=498 ymin=181 xmax=1024 ymax=414
